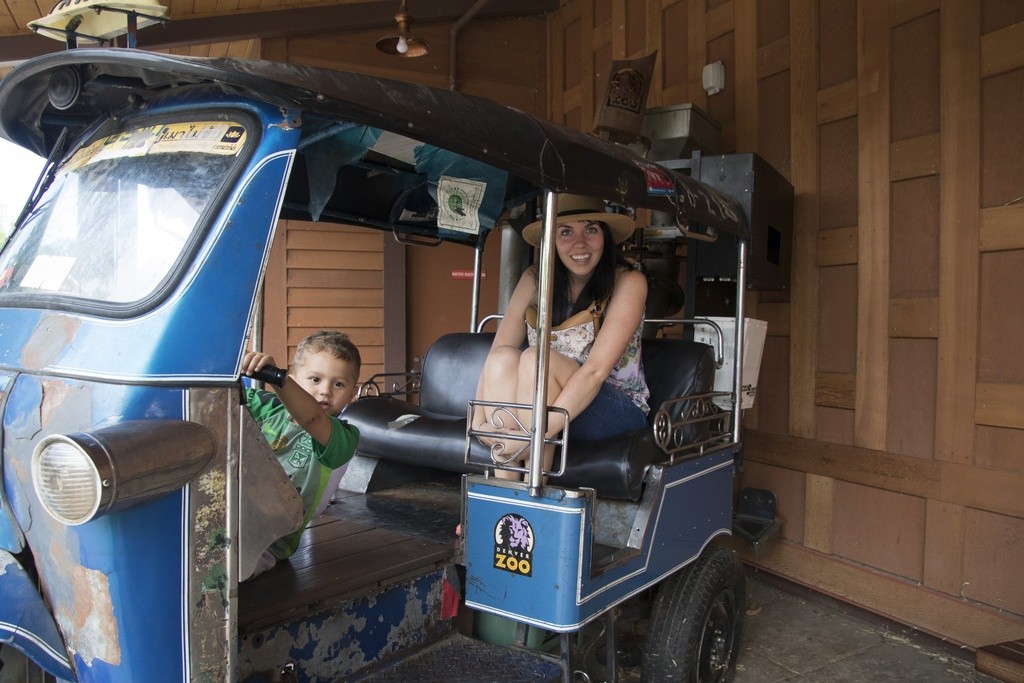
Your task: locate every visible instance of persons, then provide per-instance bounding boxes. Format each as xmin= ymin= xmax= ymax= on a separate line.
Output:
xmin=471 ymin=193 xmax=651 ymax=486
xmin=241 ymin=331 xmax=361 ymax=582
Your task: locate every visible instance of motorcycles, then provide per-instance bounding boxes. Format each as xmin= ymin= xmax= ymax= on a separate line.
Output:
xmin=0 ymin=0 xmax=796 ymax=683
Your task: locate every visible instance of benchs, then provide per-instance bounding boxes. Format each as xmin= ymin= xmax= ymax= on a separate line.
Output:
xmin=338 ymin=330 xmax=714 ymax=503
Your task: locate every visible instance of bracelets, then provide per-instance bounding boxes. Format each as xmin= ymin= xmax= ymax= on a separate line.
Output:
xmin=476 ymin=421 xmax=488 ymax=446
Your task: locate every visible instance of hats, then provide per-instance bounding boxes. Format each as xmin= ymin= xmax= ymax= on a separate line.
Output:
xmin=522 ymin=192 xmax=635 ymax=248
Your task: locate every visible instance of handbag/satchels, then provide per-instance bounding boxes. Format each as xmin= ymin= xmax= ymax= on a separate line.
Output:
xmin=526 ymin=305 xmax=599 ymax=364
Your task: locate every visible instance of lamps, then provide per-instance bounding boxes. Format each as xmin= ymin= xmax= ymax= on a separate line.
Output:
xmin=375 ymin=0 xmax=430 ymax=59
